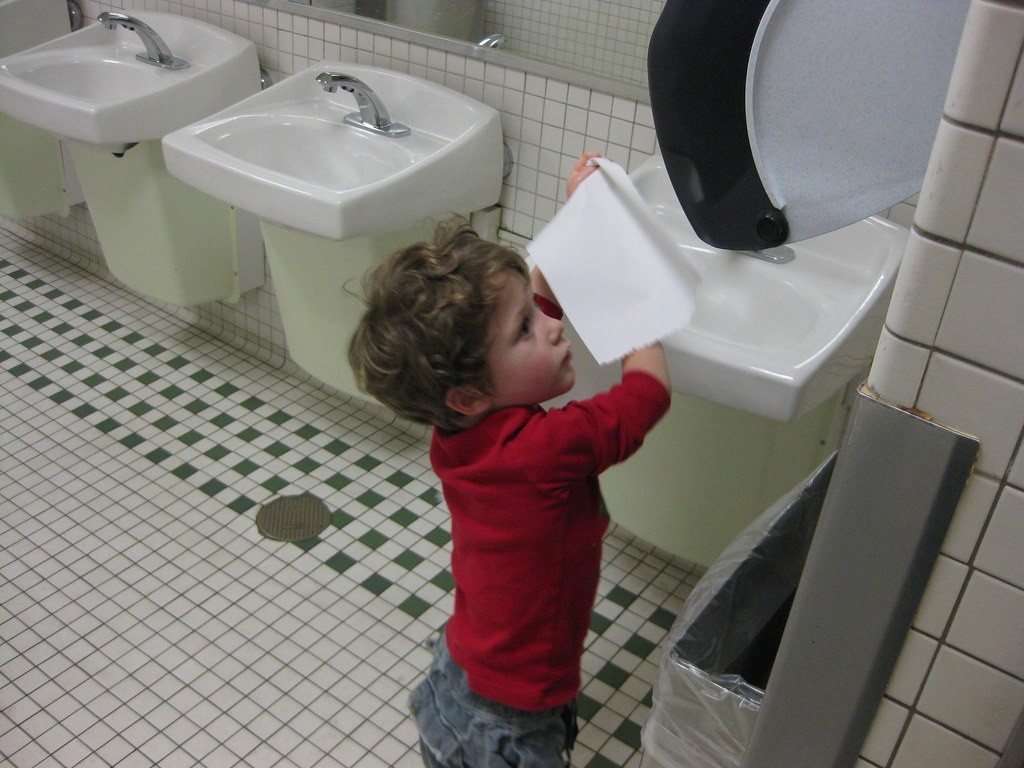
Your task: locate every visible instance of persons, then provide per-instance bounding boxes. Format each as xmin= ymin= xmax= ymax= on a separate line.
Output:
xmin=346 ymin=152 xmax=671 ymax=768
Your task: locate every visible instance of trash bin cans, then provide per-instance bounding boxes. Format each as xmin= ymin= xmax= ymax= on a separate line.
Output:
xmin=644 ymin=447 xmax=841 ymax=768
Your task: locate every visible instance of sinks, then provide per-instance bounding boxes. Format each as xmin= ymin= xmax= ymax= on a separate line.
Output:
xmin=525 ymin=153 xmax=910 ymax=423
xmin=1 ymin=9 xmax=262 ymax=145
xmin=162 ymin=61 xmax=503 ymax=242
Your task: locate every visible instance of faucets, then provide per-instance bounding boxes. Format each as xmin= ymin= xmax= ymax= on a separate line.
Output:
xmin=478 ymin=33 xmax=506 ymax=49
xmin=315 ymin=71 xmax=411 ymax=138
xmin=98 ymin=12 xmax=190 ymax=71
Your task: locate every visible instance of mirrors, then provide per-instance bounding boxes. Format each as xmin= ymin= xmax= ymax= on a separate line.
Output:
xmin=245 ymin=0 xmax=666 ymax=103
